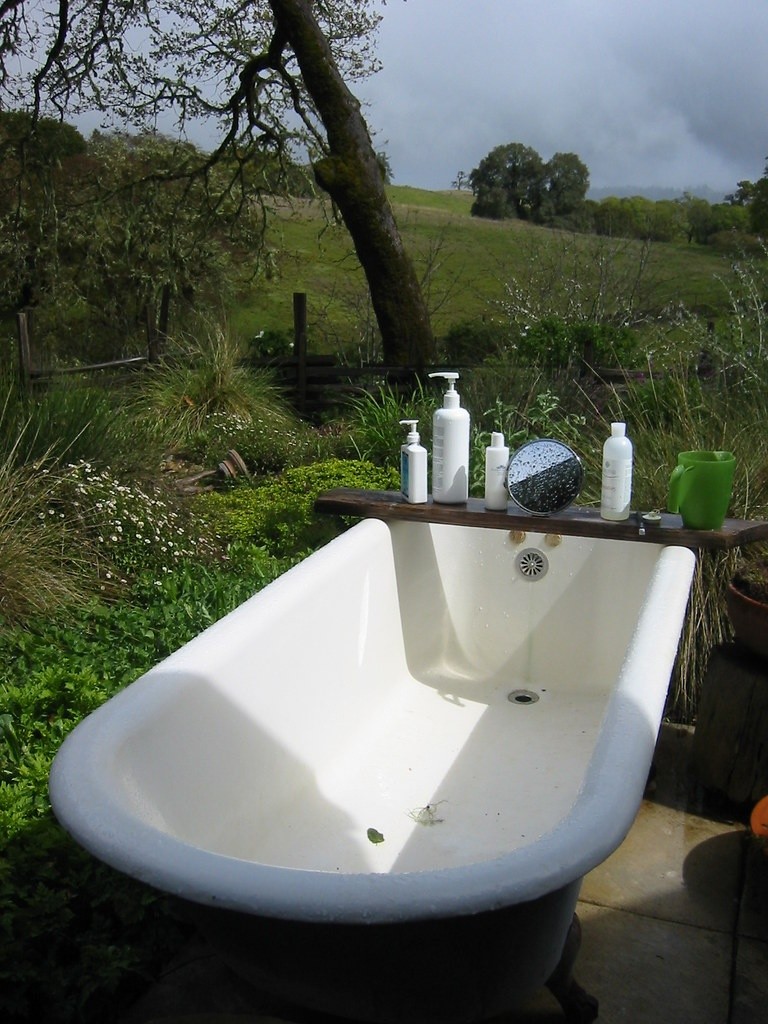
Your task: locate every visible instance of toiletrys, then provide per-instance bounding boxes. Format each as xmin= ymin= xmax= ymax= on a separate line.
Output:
xmin=484 ymin=431 xmax=510 ymax=511
xmin=428 ymin=372 xmax=470 ymax=504
xmin=399 ymin=419 xmax=428 ymax=504
xmin=601 ymin=422 xmax=632 ymax=521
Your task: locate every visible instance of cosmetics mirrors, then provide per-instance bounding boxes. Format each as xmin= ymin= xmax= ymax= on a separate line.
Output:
xmin=506 ymin=439 xmax=584 ymax=516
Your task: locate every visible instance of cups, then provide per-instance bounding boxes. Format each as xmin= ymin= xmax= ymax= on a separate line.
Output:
xmin=667 ymin=451 xmax=737 ymax=530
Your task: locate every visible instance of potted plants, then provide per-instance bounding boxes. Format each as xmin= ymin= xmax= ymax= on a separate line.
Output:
xmin=726 ymin=551 xmax=768 ymax=661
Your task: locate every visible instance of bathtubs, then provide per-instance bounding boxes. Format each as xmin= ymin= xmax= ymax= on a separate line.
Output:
xmin=48 ymin=520 xmax=698 ymax=926
